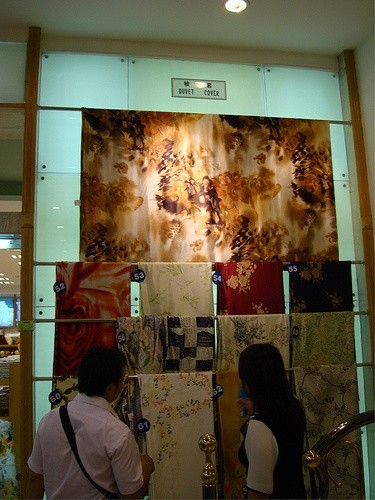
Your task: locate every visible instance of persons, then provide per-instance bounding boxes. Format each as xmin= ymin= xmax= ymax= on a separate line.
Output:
xmin=237 ymin=342 xmax=306 ymax=500
xmin=27 ymin=345 xmax=155 ymax=500
